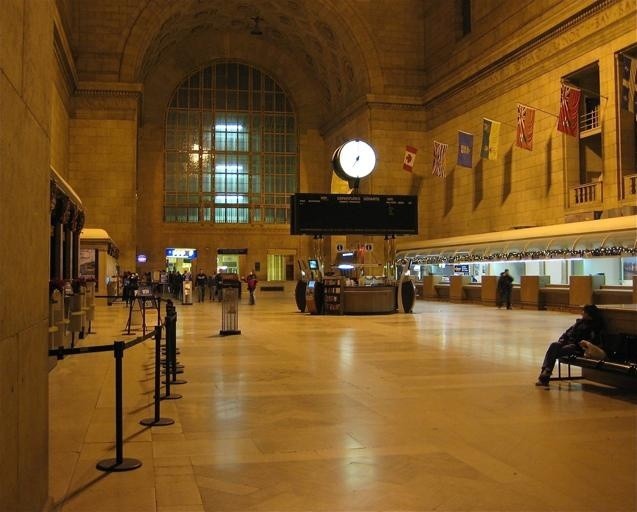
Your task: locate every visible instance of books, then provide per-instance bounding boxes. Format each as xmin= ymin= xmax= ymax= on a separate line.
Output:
xmin=322 ymin=277 xmax=342 ymax=313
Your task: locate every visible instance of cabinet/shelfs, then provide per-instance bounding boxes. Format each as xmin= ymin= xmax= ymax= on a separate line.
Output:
xmin=321 ymin=279 xmax=344 ymax=315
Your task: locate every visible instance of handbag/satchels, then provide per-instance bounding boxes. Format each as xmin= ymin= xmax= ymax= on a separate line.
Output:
xmin=578 ymin=339 xmax=607 ymax=360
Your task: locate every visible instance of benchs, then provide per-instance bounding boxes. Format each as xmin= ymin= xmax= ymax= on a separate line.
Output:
xmin=550 ymin=332 xmax=637 ymax=381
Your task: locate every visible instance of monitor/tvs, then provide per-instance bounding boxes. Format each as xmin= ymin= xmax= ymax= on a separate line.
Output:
xmin=137 ymin=254 xmax=148 ymax=263
xmin=309 ymin=259 xmax=318 ymax=269
xmin=308 ymin=280 xmax=314 ymax=287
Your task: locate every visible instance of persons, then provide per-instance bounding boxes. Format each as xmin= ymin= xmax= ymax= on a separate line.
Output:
xmin=499 ymin=269 xmax=513 ymax=310
xmin=498 ymin=271 xmax=506 ymax=307
xmin=533 ymin=303 xmax=603 ymax=388
xmin=118 ymin=265 xmax=258 ymax=308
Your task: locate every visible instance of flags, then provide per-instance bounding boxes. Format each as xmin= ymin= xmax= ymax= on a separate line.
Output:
xmin=430 ymin=140 xmax=449 ymax=179
xmin=619 ymin=52 xmax=637 ymax=114
xmin=513 ymin=102 xmax=535 ymax=152
xmin=401 ymin=144 xmax=418 ymax=174
xmin=555 ymin=80 xmax=581 ymax=138
xmin=478 ymin=117 xmax=501 ymax=163
xmin=456 ymin=129 xmax=473 ymax=169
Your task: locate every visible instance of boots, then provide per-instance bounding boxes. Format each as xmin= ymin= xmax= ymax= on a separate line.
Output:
xmin=534 ymin=366 xmax=552 ymax=388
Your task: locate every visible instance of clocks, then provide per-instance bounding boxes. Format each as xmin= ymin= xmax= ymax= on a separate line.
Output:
xmin=332 ymin=140 xmax=378 ymax=180
